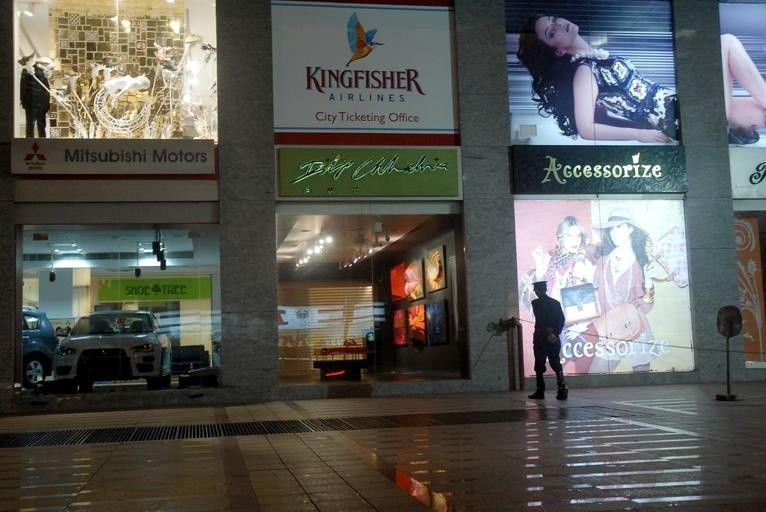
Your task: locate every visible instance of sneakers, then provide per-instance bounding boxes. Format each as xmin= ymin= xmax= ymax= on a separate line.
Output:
xmin=556 ymin=386 xmax=568 ymax=400
xmin=528 ymin=391 xmax=544 ymax=399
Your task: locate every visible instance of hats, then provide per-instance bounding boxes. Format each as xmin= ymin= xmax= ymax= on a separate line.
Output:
xmin=592 ymin=210 xmax=633 ymax=239
xmin=531 ymin=281 xmax=547 ymax=290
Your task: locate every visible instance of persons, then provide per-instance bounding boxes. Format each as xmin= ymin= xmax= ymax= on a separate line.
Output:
xmin=516 ymin=12 xmax=766 ymax=144
xmin=587 ymin=208 xmax=689 ymax=374
xmin=20 ymin=65 xmax=50 ymax=137
xmin=519 ymin=215 xmax=600 ymax=375
xmin=528 ymin=281 xmax=568 ymax=400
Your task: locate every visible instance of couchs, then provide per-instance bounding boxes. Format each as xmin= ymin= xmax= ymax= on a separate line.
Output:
xmin=172 ymin=344 xmax=208 ymax=372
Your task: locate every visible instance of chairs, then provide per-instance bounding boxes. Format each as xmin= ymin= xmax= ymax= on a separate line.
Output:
xmin=90 ymin=320 xmax=143 ymax=332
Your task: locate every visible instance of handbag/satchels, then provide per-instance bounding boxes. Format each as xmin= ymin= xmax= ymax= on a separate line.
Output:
xmin=597 ymin=302 xmax=645 ymax=348
xmin=560 ymin=283 xmax=601 ymax=325
xmin=650 ymin=225 xmax=688 ymax=287
xmin=518 ymin=268 xmax=540 ymax=315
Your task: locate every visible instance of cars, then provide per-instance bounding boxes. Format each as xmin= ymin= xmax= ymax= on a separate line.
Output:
xmin=52 ymin=309 xmax=173 ymax=394
xmin=21 ymin=305 xmax=59 ymax=390
